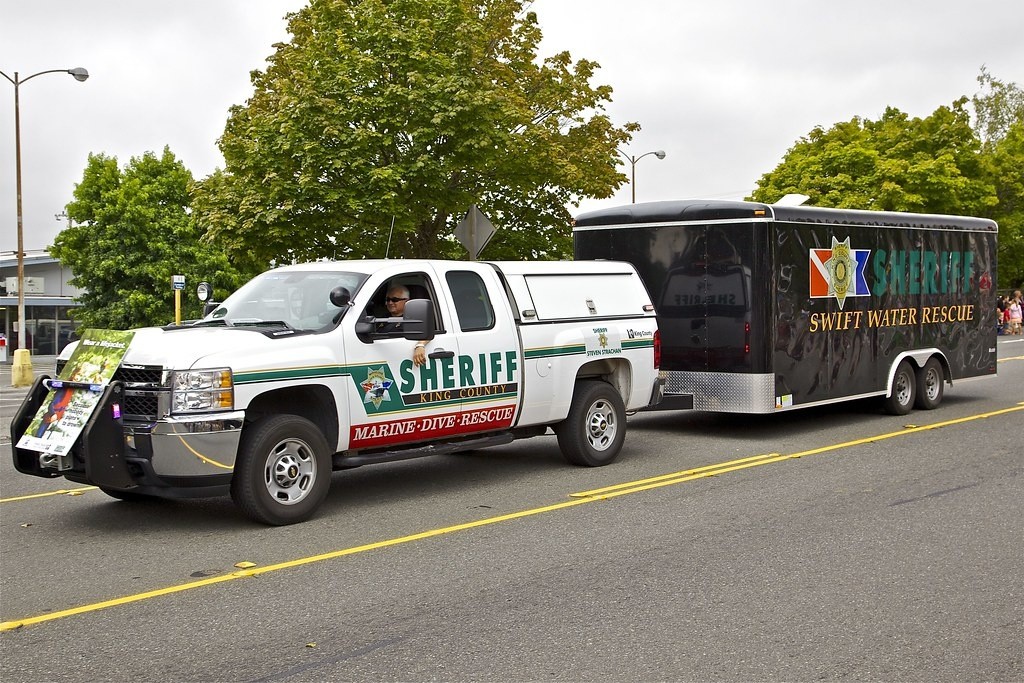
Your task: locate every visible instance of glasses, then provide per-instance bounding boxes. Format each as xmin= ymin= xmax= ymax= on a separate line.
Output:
xmin=386 ymin=297 xmax=407 ymax=303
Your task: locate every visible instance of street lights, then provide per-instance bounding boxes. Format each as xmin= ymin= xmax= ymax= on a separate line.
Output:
xmin=632 ymin=149 xmax=666 ymax=204
xmin=12 ymin=66 xmax=90 ymax=385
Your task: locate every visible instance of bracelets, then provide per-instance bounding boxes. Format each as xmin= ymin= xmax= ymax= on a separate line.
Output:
xmin=414 ymin=344 xmax=426 ymax=349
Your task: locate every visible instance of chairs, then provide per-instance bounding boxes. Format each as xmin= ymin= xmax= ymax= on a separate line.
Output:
xmin=380 ymin=283 xmax=437 ymax=332
xmin=452 ymin=283 xmax=487 ymax=327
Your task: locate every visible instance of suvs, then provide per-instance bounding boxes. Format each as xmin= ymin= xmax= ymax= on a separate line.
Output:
xmin=9 ymin=257 xmax=667 ymax=528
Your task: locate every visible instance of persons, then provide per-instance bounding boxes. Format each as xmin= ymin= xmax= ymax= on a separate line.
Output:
xmin=374 ymin=283 xmax=433 ymax=367
xmin=997 ymin=291 xmax=1024 ymax=335
